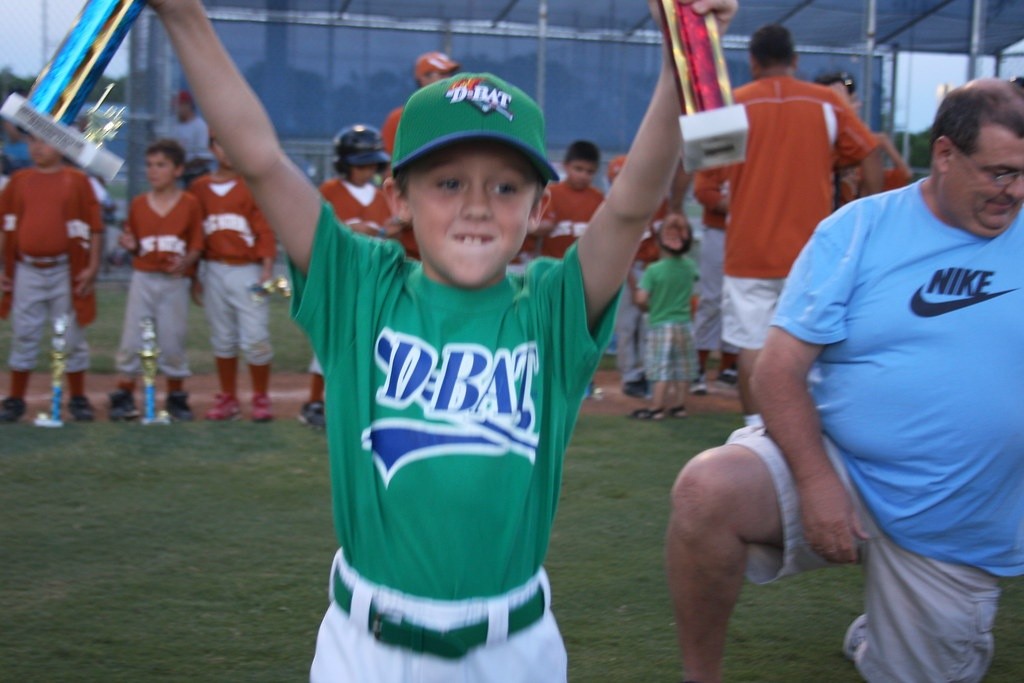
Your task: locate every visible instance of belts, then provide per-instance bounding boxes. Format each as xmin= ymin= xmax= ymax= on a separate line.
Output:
xmin=18 ymin=256 xmax=71 ymax=269
xmin=329 ymin=574 xmax=544 ymax=659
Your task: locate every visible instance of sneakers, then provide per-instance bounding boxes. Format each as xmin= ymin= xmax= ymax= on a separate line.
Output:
xmin=204 ymin=391 xmax=242 ymax=422
xmin=298 ymin=399 xmax=326 ymax=429
xmin=0 ymin=397 xmax=26 ymax=421
xmin=68 ymin=395 xmax=97 ymax=422
xmin=251 ymin=395 xmax=275 ymax=422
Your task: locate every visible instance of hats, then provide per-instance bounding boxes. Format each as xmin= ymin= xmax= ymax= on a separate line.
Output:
xmin=393 ymin=72 xmax=561 ymax=184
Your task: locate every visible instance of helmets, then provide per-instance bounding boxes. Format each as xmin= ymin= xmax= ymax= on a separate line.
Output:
xmin=331 ymin=125 xmax=392 ymax=167
xmin=411 ymin=52 xmax=458 ymax=82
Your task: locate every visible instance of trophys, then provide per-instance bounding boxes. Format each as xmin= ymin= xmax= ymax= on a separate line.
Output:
xmin=36 ymin=319 xmax=64 ymax=428
xmin=0 ymin=0 xmax=150 ymax=186
xmin=139 ymin=316 xmax=170 ymax=425
xmin=250 ymin=276 xmax=290 ymax=304
xmin=659 ymin=0 xmax=749 ymax=174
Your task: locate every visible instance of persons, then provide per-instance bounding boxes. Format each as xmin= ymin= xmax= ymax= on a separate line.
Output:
xmin=141 ymin=0 xmax=740 ymax=683
xmin=382 ymin=23 xmax=915 ymax=422
xmin=668 ymin=78 xmax=1024 ymax=683
xmin=189 ymin=131 xmax=277 ymax=423
xmin=110 ymin=140 xmax=203 ymax=421
xmin=299 ymin=124 xmax=405 ymax=428
xmin=152 ymin=90 xmax=213 ymax=184
xmin=0 ymin=90 xmax=105 ymax=423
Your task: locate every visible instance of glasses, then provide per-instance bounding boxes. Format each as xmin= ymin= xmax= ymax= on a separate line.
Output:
xmin=956 ymin=151 xmax=1024 ymax=187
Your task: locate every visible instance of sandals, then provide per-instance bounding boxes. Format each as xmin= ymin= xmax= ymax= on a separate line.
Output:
xmin=626 ymin=408 xmax=665 ymax=420
xmin=668 ymin=406 xmax=688 ymax=419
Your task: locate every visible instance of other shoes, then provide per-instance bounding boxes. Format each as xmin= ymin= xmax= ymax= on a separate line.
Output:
xmin=164 ymin=389 xmax=194 ymax=421
xmin=688 ymin=378 xmax=707 ymax=394
xmin=624 ymin=379 xmax=646 ymax=398
xmin=118 ymin=390 xmax=141 ymax=418
